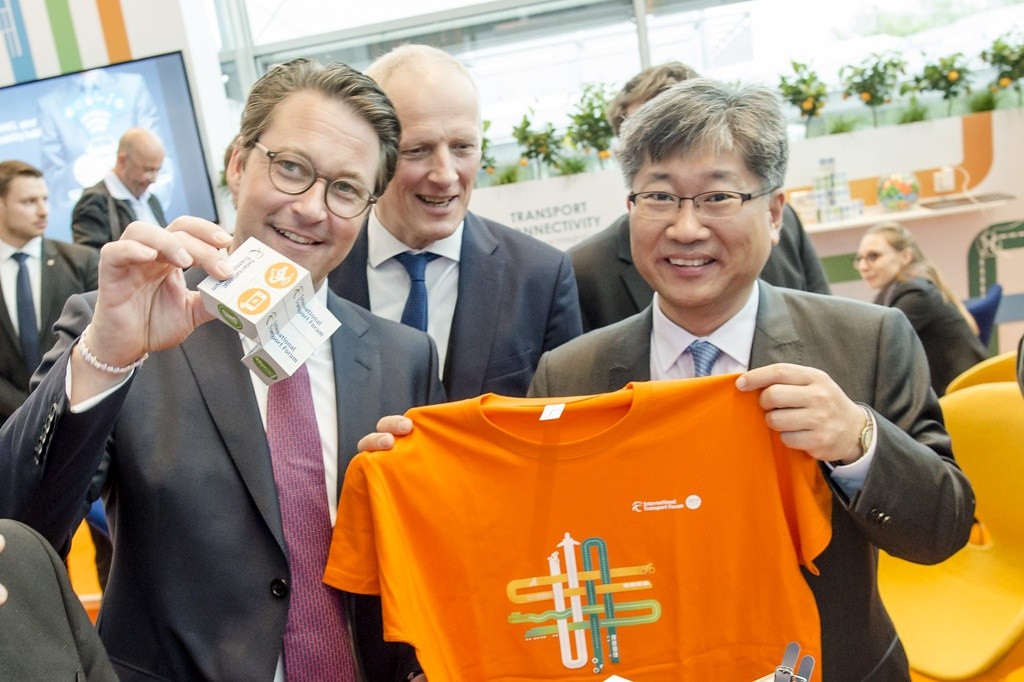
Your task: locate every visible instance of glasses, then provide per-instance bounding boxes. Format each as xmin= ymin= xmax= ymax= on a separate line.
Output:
xmin=853 ymin=249 xmax=887 ymax=270
xmin=254 ymin=141 xmax=377 ymax=220
xmin=629 ymin=182 xmax=782 ymax=218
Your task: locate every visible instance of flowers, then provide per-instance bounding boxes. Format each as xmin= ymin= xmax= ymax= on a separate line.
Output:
xmin=916 ymin=51 xmax=973 ymax=119
xmin=474 ymin=120 xmax=496 ymax=189
xmin=842 ymin=52 xmax=906 ymax=128
xmin=980 ymin=31 xmax=1024 ymax=109
xmin=512 ymin=114 xmax=563 ymax=180
xmin=565 ymin=85 xmax=622 ymax=170
xmin=779 ymin=61 xmax=825 ymax=140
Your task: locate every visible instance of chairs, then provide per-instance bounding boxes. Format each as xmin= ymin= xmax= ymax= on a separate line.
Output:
xmin=880 ymin=349 xmax=1024 ymax=682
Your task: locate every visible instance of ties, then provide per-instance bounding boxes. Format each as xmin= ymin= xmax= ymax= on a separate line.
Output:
xmin=393 ymin=252 xmax=443 ymax=333
xmin=690 ymin=341 xmax=722 ymax=378
xmin=265 ymin=362 xmax=357 ymax=682
xmin=12 ymin=253 xmax=40 ymax=374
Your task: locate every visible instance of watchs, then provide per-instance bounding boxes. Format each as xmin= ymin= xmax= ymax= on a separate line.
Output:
xmin=858 ymin=404 xmax=874 ymax=456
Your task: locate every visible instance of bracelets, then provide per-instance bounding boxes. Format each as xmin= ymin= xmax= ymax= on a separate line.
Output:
xmin=78 ymin=323 xmax=148 ymax=375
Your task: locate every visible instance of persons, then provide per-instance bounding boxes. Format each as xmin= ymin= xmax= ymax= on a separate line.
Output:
xmin=0 ymin=158 xmax=113 ymax=602
xmin=852 ymin=221 xmax=992 ymax=399
xmin=565 ymin=61 xmax=833 ymax=334
xmin=0 ymin=56 xmax=450 ymax=682
xmin=328 ymin=40 xmax=584 ymax=403
xmin=356 ymin=78 xmax=975 ymax=682
xmin=71 ymin=126 xmax=169 ymax=250
xmin=0 ymin=518 xmax=120 ymax=682
